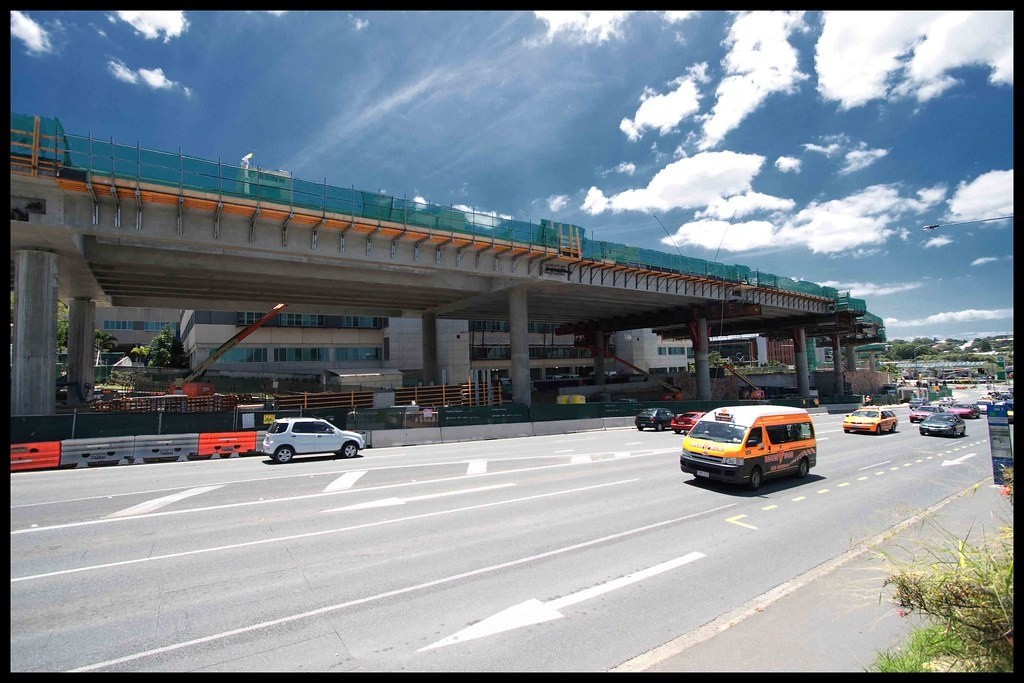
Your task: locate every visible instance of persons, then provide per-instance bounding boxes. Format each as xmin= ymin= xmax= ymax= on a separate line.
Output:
xmin=866 ymin=396 xmax=872 ymax=405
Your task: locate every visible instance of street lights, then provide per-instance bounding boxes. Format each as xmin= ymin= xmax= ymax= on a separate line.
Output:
xmin=913 ymin=343 xmax=935 ymax=370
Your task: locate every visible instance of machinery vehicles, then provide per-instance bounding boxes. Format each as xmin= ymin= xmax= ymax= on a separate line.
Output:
xmin=573 ymin=343 xmax=683 ymax=401
xmin=723 ymin=362 xmax=765 ymax=400
xmin=168 ymin=301 xmax=291 ymax=395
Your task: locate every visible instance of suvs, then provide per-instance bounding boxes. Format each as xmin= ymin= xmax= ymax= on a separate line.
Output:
xmin=261 ymin=416 xmax=365 ymax=464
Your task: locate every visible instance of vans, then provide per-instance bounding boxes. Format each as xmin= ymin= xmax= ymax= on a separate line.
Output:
xmin=680 ymin=404 xmax=816 ymax=491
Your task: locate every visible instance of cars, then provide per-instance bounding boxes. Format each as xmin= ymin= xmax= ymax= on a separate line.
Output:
xmin=975 ymin=387 xmax=1014 ymax=425
xmin=842 ymin=406 xmax=899 ymax=436
xmin=946 ymin=402 xmax=980 ymax=420
xmin=909 ymin=398 xmax=931 ymax=410
xmin=670 ymin=412 xmax=708 ymax=434
xmin=930 ymin=396 xmax=960 ymax=412
xmin=635 ymin=408 xmax=677 ymax=432
xmin=909 ymin=405 xmax=945 ymax=424
xmin=918 ymin=411 xmax=966 ymax=438
